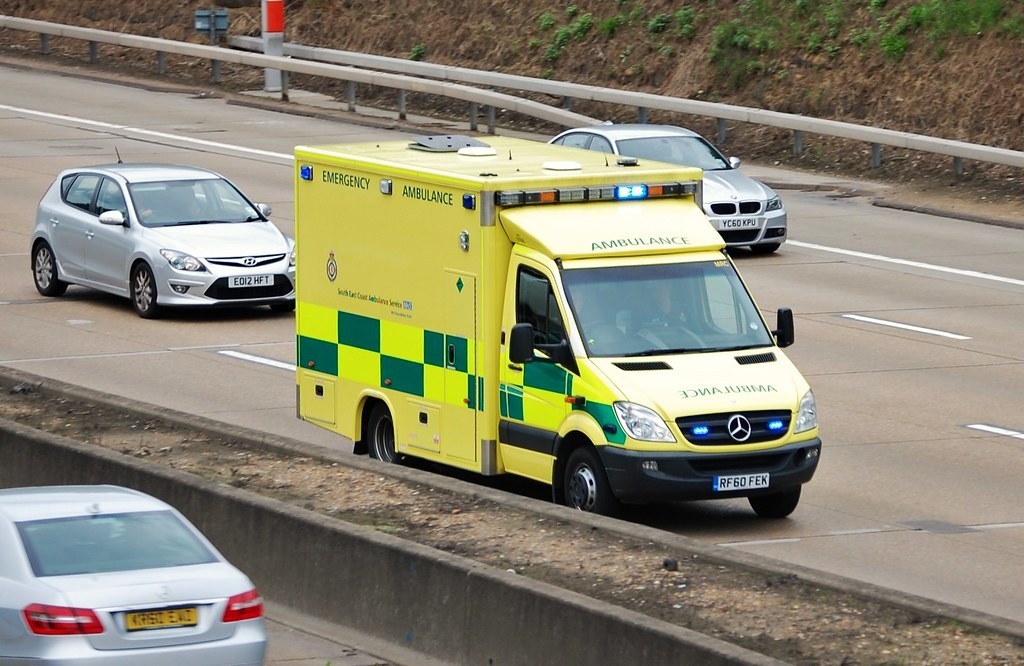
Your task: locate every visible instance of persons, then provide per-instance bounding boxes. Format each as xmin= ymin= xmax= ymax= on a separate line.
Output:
xmin=626 ymin=282 xmax=703 ymax=341
xmin=121 ymin=193 xmax=152 ymax=220
xmin=552 ymin=291 xmax=596 ymax=345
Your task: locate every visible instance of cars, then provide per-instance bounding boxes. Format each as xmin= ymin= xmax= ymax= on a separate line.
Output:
xmin=0 ymin=485 xmax=269 ymax=666
xmin=547 ymin=121 xmax=787 ymax=257
xmin=30 ymin=157 xmax=296 ymax=319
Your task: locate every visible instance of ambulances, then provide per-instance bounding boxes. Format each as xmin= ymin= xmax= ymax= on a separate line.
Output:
xmin=294 ymin=133 xmax=822 ymax=517
